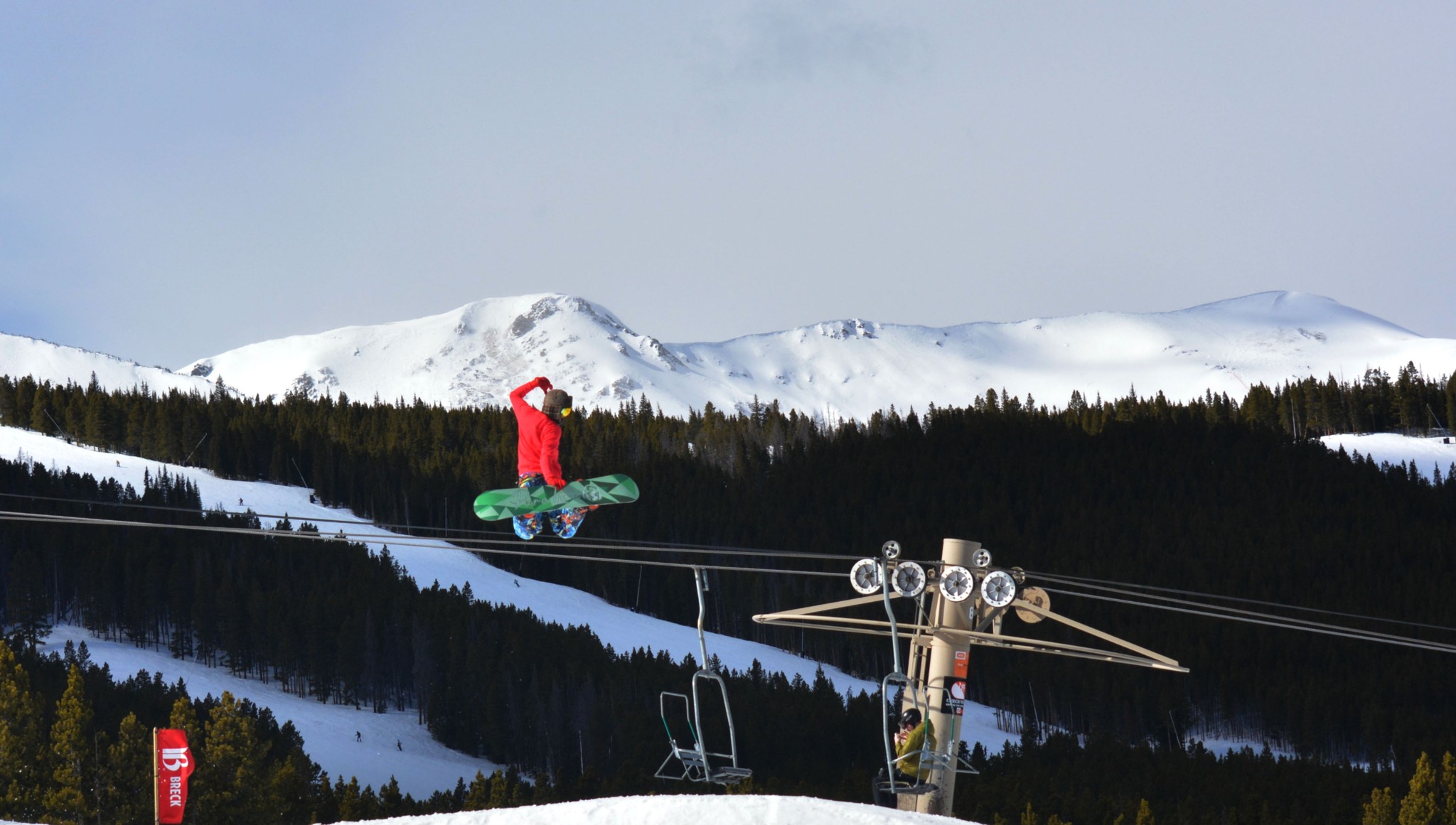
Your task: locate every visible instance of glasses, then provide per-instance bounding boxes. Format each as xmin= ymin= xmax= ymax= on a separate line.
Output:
xmin=559 ymin=407 xmax=573 ymax=419
xmin=898 ymin=722 xmax=906 ymax=729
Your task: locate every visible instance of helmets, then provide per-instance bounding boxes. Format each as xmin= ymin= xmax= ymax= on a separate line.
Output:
xmin=902 ymin=709 xmax=920 ymax=725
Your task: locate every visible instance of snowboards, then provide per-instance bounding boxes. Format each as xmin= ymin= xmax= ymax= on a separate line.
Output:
xmin=473 ymin=473 xmax=639 ymax=521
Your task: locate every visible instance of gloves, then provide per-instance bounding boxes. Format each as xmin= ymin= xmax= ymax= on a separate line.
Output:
xmin=546 ymin=475 xmax=566 ymax=490
xmin=535 ymin=376 xmax=553 ymax=394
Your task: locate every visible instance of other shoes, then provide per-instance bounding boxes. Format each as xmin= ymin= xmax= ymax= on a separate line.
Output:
xmin=577 ymin=477 xmax=599 ymax=510
xmin=523 ymin=513 xmax=533 ymax=519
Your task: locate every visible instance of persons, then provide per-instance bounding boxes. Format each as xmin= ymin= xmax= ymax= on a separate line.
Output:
xmin=239 ymin=498 xmax=243 ymax=506
xmin=510 ymin=377 xmax=600 ymax=540
xmin=117 ymin=461 xmax=120 ymax=467
xmin=871 ymin=707 xmax=937 ymax=809
xmin=514 ymin=579 xmax=520 ymax=587
xmin=397 ymin=739 xmax=401 ymax=751
xmin=355 ymin=731 xmax=361 ymax=742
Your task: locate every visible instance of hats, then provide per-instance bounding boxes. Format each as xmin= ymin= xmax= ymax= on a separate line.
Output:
xmin=542 ymin=388 xmax=569 ymax=418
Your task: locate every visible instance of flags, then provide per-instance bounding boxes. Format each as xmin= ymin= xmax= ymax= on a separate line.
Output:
xmin=156 ymin=728 xmax=195 ymax=824
